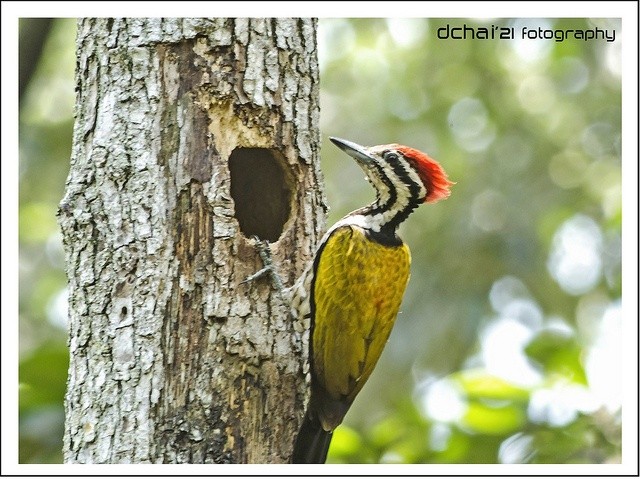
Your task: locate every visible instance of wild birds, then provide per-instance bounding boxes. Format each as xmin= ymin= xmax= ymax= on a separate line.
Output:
xmin=238 ymin=134 xmax=458 ymax=464
